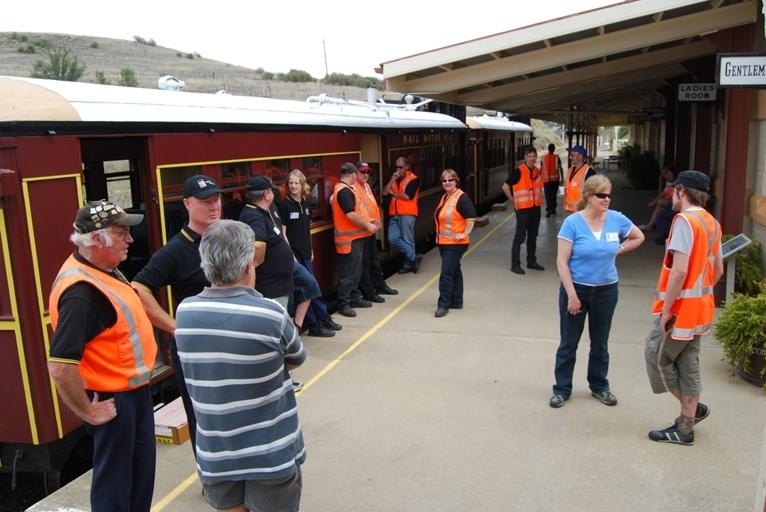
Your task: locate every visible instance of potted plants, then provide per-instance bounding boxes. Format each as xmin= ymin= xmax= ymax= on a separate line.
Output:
xmin=714 ymin=280 xmax=766 ymax=388
xmin=712 ymin=232 xmax=765 ymax=308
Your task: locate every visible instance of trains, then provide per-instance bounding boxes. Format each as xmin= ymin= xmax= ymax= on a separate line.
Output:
xmin=0 ymin=72 xmax=532 ymax=504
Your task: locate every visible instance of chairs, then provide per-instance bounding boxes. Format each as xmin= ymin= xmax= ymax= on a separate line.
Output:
xmin=588 ymin=155 xmax=623 ymax=172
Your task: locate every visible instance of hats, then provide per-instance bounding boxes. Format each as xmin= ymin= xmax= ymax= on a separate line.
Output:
xmin=340 ymin=162 xmax=356 ymax=172
xmin=566 ymin=145 xmax=587 ymax=155
xmin=355 ymin=161 xmax=371 ymax=170
xmin=183 ymin=175 xmax=224 ymax=200
xmin=246 ymin=175 xmax=273 ymax=191
xmin=667 ymin=170 xmax=711 ymax=192
xmin=74 ymin=200 xmax=144 ymax=234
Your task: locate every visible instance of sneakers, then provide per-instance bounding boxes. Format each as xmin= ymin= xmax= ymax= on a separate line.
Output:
xmin=398 ymin=256 xmax=422 ymax=274
xmin=435 ymin=307 xmax=449 ymax=317
xmin=591 ymin=389 xmax=617 ymax=406
xmin=549 ymin=393 xmax=569 ymax=408
xmin=290 ymin=380 xmax=304 ymax=393
xmin=338 ymin=307 xmax=357 ymax=317
xmin=376 ymin=285 xmax=398 ymax=295
xmin=363 ymin=294 xmax=385 ymax=303
xmin=325 ymin=321 xmax=342 ymax=330
xmin=350 ymin=300 xmax=372 ymax=308
xmin=449 ymin=302 xmax=464 ymax=309
xmin=526 ymin=262 xmax=544 ymax=271
xmin=672 ymin=402 xmax=710 ymax=425
xmin=648 ymin=425 xmax=696 ymax=446
xmin=510 ymin=267 xmax=525 ymax=274
xmin=308 ymin=327 xmax=336 ymax=337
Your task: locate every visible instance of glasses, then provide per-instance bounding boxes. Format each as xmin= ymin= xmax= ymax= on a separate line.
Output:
xmin=591 ymin=192 xmax=611 ymax=198
xmin=395 ymin=166 xmax=405 ymax=169
xmin=441 ymin=178 xmax=455 ymax=182
xmin=360 ymin=170 xmax=370 ymax=174
